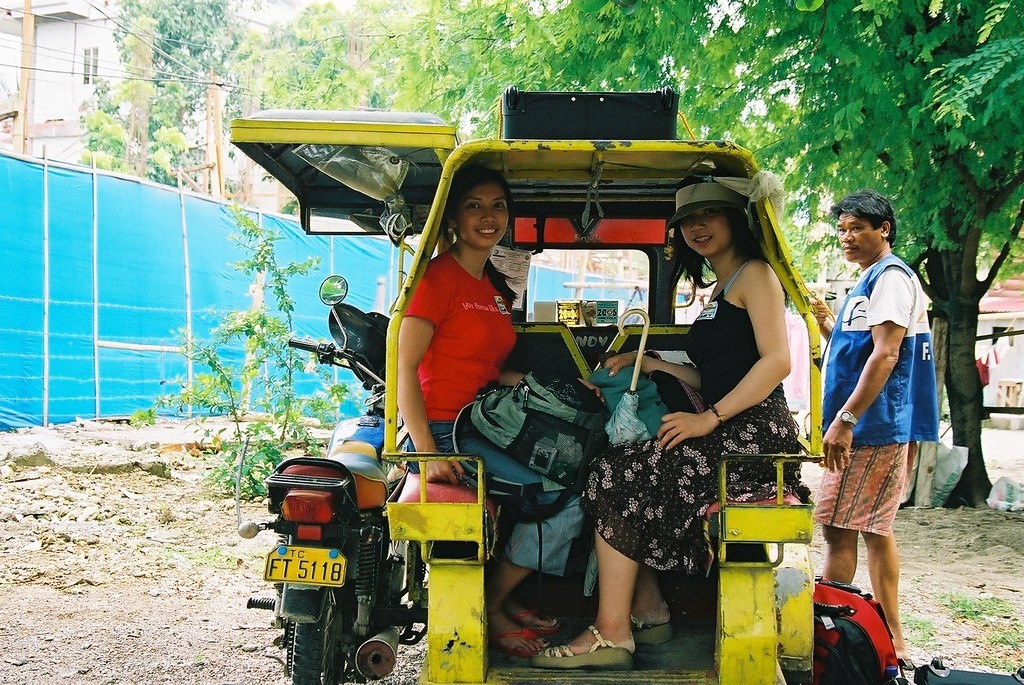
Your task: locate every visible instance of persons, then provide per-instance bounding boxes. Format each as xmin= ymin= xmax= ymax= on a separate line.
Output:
xmin=397 ymin=165 xmax=586 ymax=658
xmin=814 ymin=190 xmax=939 ymax=670
xmin=529 ymin=176 xmax=802 ymax=671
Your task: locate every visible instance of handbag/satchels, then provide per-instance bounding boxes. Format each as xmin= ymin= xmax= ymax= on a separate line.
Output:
xmin=915 ymin=442 xmax=968 ymax=508
xmin=899 ymin=441 xmax=922 ymax=504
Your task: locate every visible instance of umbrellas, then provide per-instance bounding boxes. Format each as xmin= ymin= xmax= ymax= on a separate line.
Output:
xmin=584 ymin=309 xmax=650 ymax=614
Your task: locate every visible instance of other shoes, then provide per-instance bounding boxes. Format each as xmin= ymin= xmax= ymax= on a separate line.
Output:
xmin=901 ymin=658 xmax=915 ymax=671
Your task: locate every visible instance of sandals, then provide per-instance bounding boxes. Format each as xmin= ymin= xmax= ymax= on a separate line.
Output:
xmin=629 ymin=614 xmax=676 ymax=644
xmin=531 ymin=625 xmax=634 ymax=667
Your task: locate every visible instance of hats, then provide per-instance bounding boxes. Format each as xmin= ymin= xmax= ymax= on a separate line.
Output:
xmin=666 ymin=171 xmax=745 ymax=226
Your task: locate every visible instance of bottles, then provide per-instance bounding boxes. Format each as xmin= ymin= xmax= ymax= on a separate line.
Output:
xmin=884 ymin=665 xmax=900 ymax=685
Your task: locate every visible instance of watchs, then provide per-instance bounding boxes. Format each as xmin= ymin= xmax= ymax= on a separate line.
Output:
xmin=836 ymin=409 xmax=859 ymax=426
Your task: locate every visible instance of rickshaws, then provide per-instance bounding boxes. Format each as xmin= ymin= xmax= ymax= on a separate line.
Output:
xmin=228 ymin=83 xmax=828 ymax=685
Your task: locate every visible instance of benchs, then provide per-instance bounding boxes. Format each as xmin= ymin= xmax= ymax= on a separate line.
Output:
xmin=702 ymin=432 xmax=826 ymax=568
xmin=383 ymin=424 xmax=501 ymax=561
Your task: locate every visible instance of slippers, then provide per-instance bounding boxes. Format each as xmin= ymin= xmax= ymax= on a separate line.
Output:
xmin=512 ymin=610 xmax=561 ymax=634
xmin=487 ymin=628 xmax=546 ymax=657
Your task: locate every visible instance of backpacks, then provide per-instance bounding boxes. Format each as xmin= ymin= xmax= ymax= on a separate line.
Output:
xmin=453 ymin=372 xmax=607 ymax=519
xmin=781 ymin=575 xmax=898 ymax=685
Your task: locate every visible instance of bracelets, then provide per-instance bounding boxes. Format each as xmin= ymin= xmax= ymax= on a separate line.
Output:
xmin=709 ymin=404 xmax=727 ymax=427
